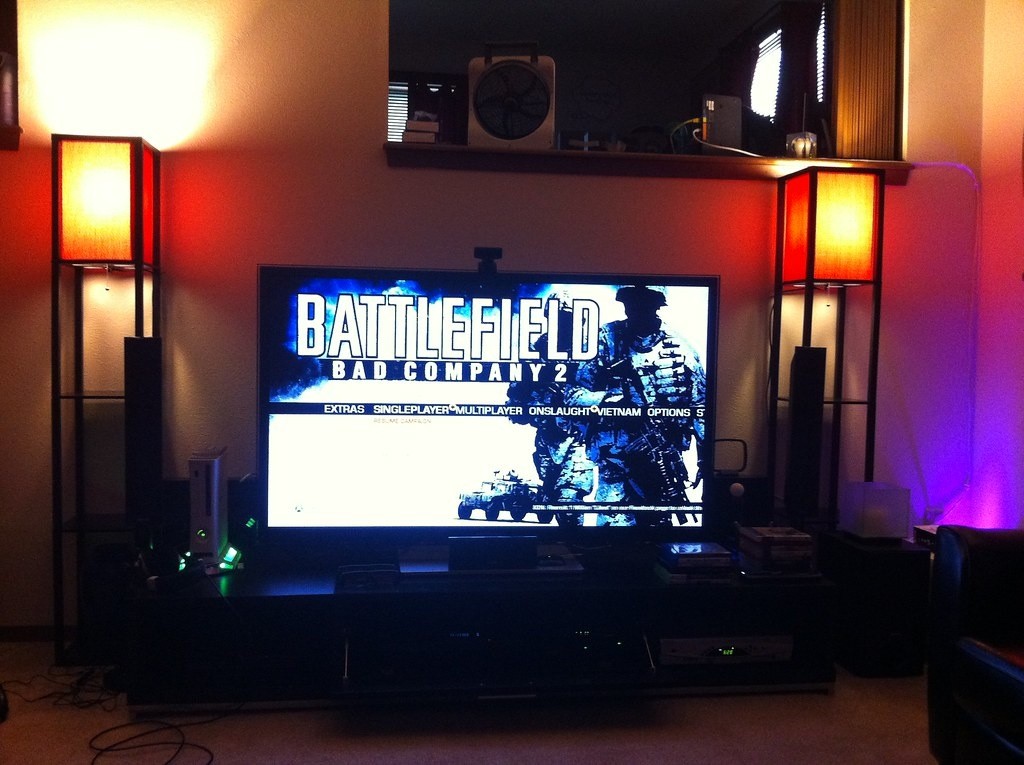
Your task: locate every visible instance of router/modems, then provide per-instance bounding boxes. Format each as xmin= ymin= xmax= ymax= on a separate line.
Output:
xmin=698 ymin=94 xmax=743 ymax=156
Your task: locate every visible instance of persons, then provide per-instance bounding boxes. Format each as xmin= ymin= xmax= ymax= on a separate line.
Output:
xmin=563 ymin=280 xmax=710 ymax=527
xmin=506 ymin=291 xmax=601 ymax=524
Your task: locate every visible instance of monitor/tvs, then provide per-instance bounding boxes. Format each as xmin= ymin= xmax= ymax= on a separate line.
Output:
xmin=254 ymin=262 xmax=720 ymax=577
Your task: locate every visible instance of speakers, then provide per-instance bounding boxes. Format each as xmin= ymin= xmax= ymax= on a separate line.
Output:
xmin=124 ymin=336 xmax=166 ymax=530
xmin=783 ymin=346 xmax=827 ymax=518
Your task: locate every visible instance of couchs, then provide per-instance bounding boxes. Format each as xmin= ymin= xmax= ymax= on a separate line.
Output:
xmin=927 ymin=527 xmax=1024 ymax=765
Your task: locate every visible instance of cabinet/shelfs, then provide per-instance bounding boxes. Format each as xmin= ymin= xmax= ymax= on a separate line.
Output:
xmin=123 ymin=565 xmax=835 ymax=716
xmin=769 ymin=166 xmax=885 ymax=528
xmin=51 ymin=134 xmax=162 ymax=668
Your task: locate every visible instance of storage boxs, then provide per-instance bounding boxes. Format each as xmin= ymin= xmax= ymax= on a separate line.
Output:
xmin=841 ymin=481 xmax=910 ymax=538
